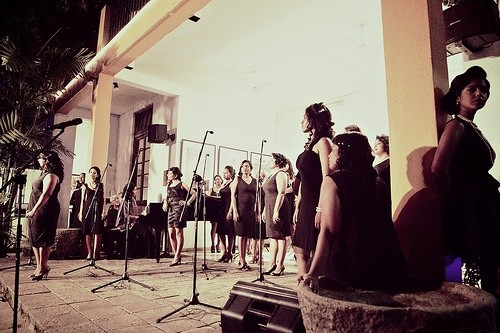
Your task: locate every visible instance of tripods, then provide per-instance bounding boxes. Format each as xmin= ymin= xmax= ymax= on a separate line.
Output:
xmin=0 ymin=247 xmax=37 ymax=271
xmin=250 ymin=179 xmax=294 ymax=291
xmin=63 ymin=174 xmax=228 ymax=324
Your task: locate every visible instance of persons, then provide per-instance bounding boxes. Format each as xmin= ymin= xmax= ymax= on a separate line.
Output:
xmin=26 ymin=150 xmax=65 ymax=282
xmin=69 ymin=102 xmax=401 ymax=294
xmin=431 ymin=66 xmax=500 ymax=302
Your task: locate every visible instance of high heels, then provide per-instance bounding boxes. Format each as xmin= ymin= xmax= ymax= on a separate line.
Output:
xmin=211 ymin=246 xmax=215 ymax=253
xmin=236 ymin=262 xmax=247 ymax=270
xmin=249 ymin=257 xmax=258 ymax=264
xmin=265 ymin=264 xmax=277 ymax=275
xmin=223 ymin=255 xmax=232 ymax=263
xmin=28 ymin=274 xmax=35 ymax=279
xmin=30 ymin=266 xmax=51 ymax=282
xmin=216 ymin=245 xmax=220 ymax=253
xmin=170 ymin=257 xmax=181 ymax=267
xmin=270 ymin=266 xmax=285 ymax=276
xmin=218 ymin=256 xmax=225 ymax=262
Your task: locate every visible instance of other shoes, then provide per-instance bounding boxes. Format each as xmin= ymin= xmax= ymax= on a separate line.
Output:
xmin=232 ymin=247 xmax=235 ymax=253
xmin=247 ymin=251 xmax=251 ymax=255
xmin=87 ymin=257 xmax=91 ymax=261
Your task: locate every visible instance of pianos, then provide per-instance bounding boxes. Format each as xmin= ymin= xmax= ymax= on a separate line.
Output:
xmin=144 ymin=199 xmax=236 ymax=264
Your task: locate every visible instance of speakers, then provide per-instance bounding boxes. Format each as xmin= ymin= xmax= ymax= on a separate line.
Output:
xmin=149 ymin=124 xmax=168 ymax=143
xmin=221 ymin=280 xmax=306 ymax=333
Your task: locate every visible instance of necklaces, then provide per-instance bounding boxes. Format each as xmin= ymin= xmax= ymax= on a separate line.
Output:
xmin=457 ymin=115 xmax=495 ymax=165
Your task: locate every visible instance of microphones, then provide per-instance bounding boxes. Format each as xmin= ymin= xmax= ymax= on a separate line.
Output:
xmin=85 ymin=162 xmax=112 ymax=219
xmin=254 ymin=140 xmax=267 ymax=212
xmin=44 ymin=118 xmax=83 ymax=131
xmin=115 ymin=148 xmax=146 ymax=227
xmin=181 ymin=131 xmax=213 ymax=222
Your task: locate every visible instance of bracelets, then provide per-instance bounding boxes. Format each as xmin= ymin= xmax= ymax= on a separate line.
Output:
xmin=315 ymin=207 xmax=322 ymax=212
